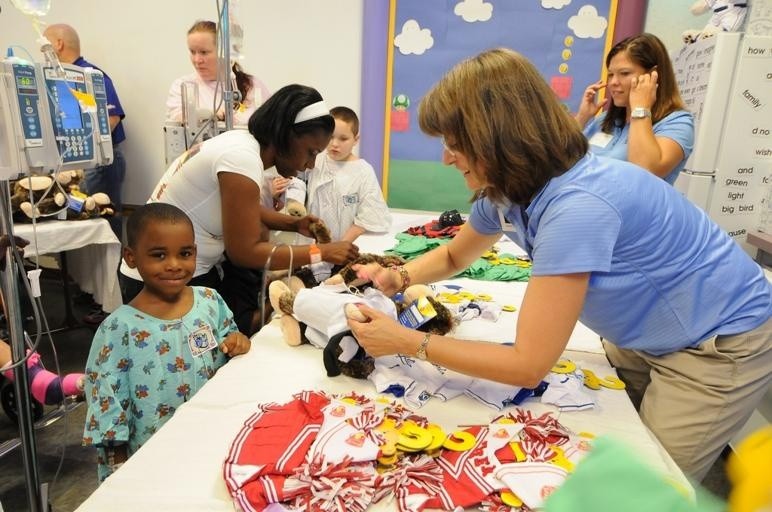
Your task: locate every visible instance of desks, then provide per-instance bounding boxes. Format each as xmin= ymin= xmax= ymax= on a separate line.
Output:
xmin=71 ymin=209 xmax=702 ymax=512
xmin=1 ymin=216 xmax=111 ymax=337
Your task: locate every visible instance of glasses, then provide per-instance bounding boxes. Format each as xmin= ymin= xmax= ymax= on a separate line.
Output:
xmin=437 ymin=138 xmax=461 ymax=158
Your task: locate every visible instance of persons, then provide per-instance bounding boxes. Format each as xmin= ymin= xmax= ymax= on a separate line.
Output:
xmin=166 ymin=20 xmax=273 ymax=125
xmin=574 ymin=31 xmax=694 ymax=185
xmin=82 ymin=203 xmax=251 ymax=482
xmin=326 ymin=46 xmax=772 ymax=485
xmin=118 ymin=84 xmax=360 ymax=339
xmin=44 ymin=24 xmax=126 ymax=242
xmin=0 ymin=339 xmax=84 ymax=406
xmin=274 ymin=108 xmax=393 ymax=282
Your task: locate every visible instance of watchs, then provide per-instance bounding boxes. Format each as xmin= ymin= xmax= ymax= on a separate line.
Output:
xmin=417 ymin=332 xmax=431 ymax=360
xmin=631 ymin=110 xmax=651 ymax=119
